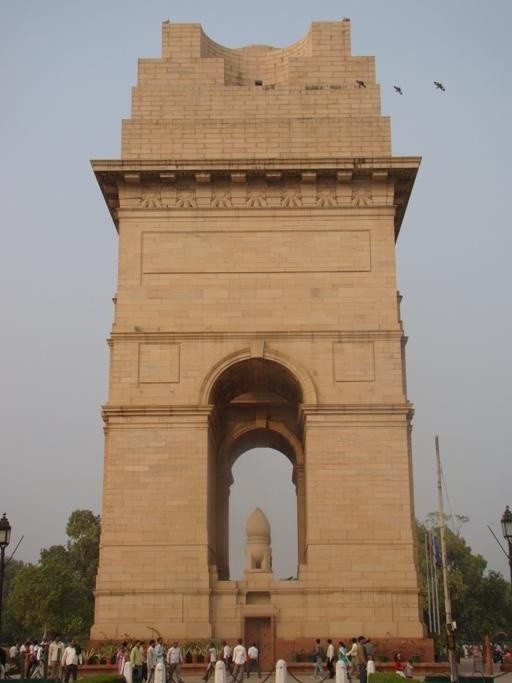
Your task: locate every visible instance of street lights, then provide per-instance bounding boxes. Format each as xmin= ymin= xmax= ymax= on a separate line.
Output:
xmin=499 ymin=503 xmax=511 ymax=601
xmin=0 ymin=510 xmax=11 ymax=616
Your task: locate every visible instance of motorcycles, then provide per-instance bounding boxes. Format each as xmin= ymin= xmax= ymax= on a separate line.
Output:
xmin=492 ymin=648 xmax=507 ymax=664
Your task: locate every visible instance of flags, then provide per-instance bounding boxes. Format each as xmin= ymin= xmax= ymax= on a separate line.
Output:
xmin=425 ymin=530 xmax=442 ymax=573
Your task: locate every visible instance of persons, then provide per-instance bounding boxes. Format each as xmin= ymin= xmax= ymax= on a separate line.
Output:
xmin=442 ymin=641 xmax=508 ymax=663
